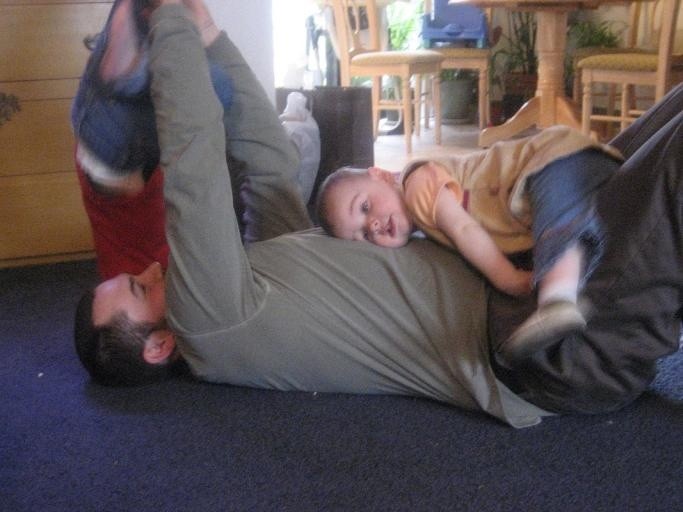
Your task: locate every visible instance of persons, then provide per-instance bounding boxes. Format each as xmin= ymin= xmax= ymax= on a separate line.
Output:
xmin=72 ymin=1 xmax=681 ymax=430
xmin=71 ymin=0 xmax=231 ymax=272
xmin=312 ymin=126 xmax=627 ymax=372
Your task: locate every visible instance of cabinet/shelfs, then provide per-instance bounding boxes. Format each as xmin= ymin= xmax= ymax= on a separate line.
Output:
xmin=0 ymin=3 xmax=114 ymax=260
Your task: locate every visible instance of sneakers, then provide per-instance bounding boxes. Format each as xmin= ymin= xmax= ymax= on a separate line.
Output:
xmin=490 ymin=299 xmax=587 ymax=366
